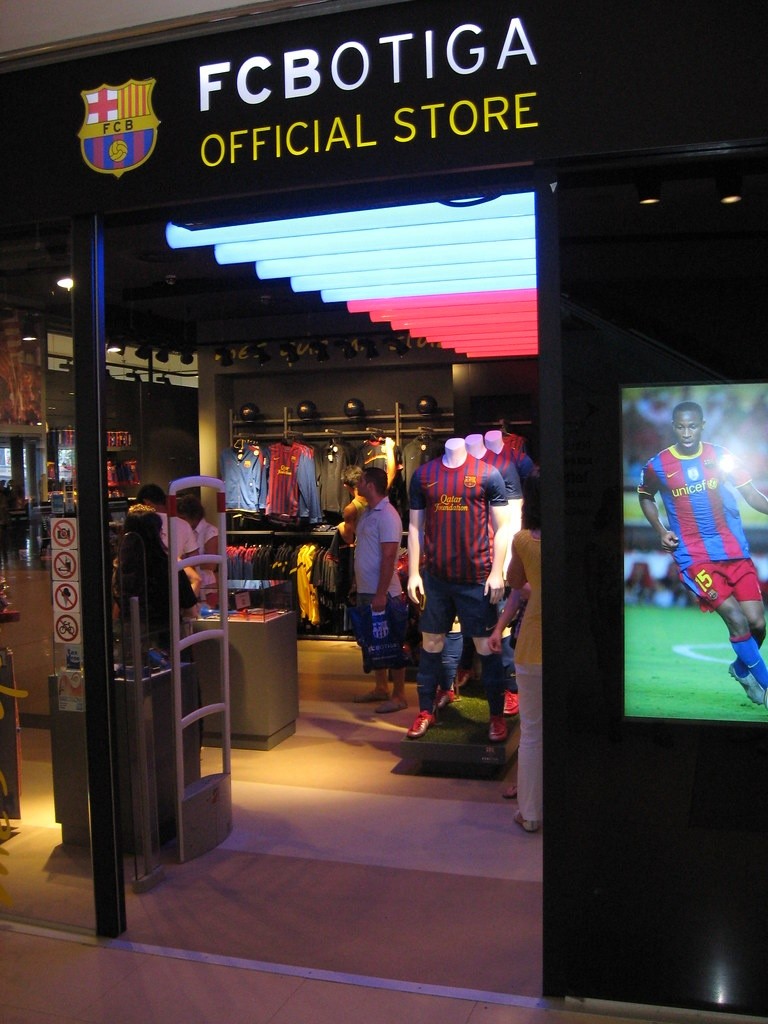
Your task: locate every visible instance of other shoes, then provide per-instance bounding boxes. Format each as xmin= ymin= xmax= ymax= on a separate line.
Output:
xmin=513 ymin=809 xmax=539 ymax=832
xmin=374 ymin=699 xmax=407 ymax=714
xmin=353 ymin=687 xmax=390 ymax=702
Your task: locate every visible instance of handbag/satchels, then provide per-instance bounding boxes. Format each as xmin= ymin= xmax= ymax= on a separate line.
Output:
xmin=347 ymin=601 xmax=414 ymax=671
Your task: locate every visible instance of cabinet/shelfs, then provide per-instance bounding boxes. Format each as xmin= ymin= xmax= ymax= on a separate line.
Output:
xmin=193 ymin=579 xmax=300 ymax=750
xmin=230 ymin=401 xmax=453 ymax=446
xmin=46 ymin=430 xmax=130 ymax=517
xmin=48 ymin=664 xmax=197 ymax=850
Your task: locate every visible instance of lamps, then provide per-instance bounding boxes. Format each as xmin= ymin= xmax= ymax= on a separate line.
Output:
xmin=105 ymin=338 xmax=409 ymax=368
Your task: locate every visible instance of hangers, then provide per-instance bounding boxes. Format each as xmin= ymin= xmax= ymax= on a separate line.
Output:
xmin=234 ymin=426 xmax=434 ymax=446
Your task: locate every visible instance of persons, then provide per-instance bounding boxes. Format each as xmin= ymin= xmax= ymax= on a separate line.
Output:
xmin=137 ymin=484 xmax=219 ymax=601
xmin=336 ymin=466 xmax=370 ymax=545
xmin=348 ymin=467 xmax=409 ymax=713
xmin=638 ymin=402 xmax=768 ymax=704
xmin=113 ymin=504 xmax=204 ymax=755
xmin=488 ymin=462 xmax=541 ymax=832
xmin=406 ymin=430 xmax=534 ymax=739
xmin=0 ymin=473 xmax=47 ymax=528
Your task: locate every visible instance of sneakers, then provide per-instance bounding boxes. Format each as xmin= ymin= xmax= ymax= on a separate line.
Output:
xmin=729 ymin=663 xmax=766 ymax=705
xmin=502 ymin=691 xmax=519 ymax=715
xmin=489 ymin=714 xmax=507 ymax=742
xmin=432 ymin=687 xmax=455 ymax=709
xmin=407 ymin=710 xmax=435 ymax=738
